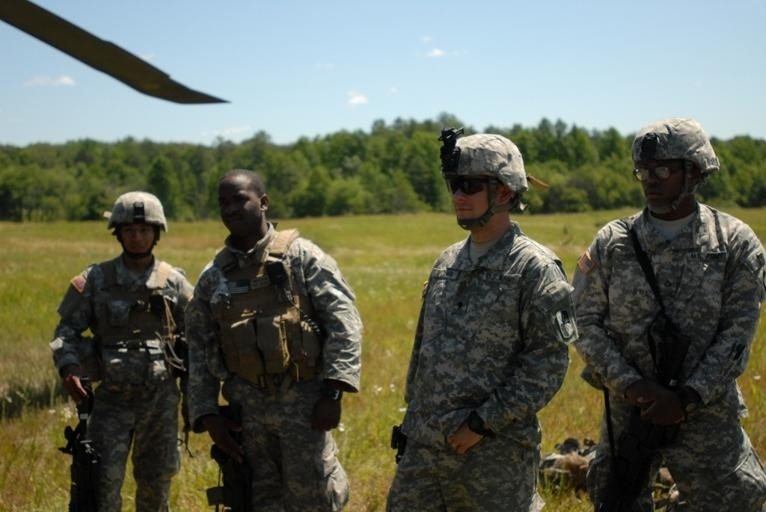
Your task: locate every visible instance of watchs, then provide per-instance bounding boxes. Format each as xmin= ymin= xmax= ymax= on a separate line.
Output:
xmin=468 ymin=410 xmax=489 ymax=436
xmin=676 ymin=386 xmax=697 ymax=415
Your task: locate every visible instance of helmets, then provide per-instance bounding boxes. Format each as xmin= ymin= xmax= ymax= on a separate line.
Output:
xmin=108 ymin=191 xmax=168 ymax=233
xmin=631 ymin=120 xmax=720 ymax=174
xmin=440 ymin=128 xmax=529 ymax=211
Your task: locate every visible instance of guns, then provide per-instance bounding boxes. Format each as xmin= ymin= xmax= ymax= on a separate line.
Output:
xmin=603 ymin=342 xmax=689 ymax=510
xmin=207 ymin=406 xmax=251 ymax=511
xmin=63 ymin=374 xmax=98 ymax=511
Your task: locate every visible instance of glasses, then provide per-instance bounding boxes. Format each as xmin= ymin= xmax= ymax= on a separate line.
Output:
xmin=633 ymin=166 xmax=679 ymax=181
xmin=450 ymin=178 xmax=489 ymax=195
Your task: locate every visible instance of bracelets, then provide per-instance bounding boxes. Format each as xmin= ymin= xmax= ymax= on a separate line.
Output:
xmin=319 ymin=384 xmax=342 ymax=401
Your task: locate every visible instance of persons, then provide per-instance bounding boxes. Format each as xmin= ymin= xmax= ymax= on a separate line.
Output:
xmin=386 ymin=127 xmax=580 ymax=512
xmin=571 ymin=117 xmax=766 ymax=512
xmin=184 ymin=170 xmax=364 ymax=512
xmin=50 ymin=190 xmax=195 ymax=512
